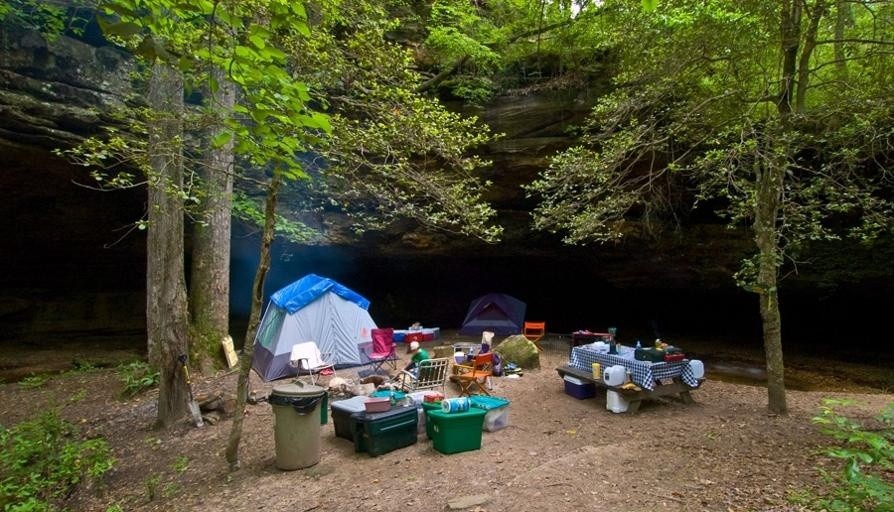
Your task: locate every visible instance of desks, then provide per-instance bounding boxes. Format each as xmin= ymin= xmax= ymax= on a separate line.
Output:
xmin=572 ymin=341 xmax=704 ymax=414
xmin=570 ymin=332 xmax=613 ymax=354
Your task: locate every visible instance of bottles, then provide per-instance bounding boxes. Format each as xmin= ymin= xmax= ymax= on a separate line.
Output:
xmin=440 ymin=397 xmax=472 ymax=413
xmin=625 ymin=367 xmax=634 ymax=383
xmin=636 ymin=340 xmax=642 ymax=350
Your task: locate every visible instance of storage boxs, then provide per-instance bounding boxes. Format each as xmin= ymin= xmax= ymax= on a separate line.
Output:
xmin=364 ymin=396 xmax=391 ymax=412
xmin=421 ymin=401 xmax=443 ymax=440
xmin=426 ymin=407 xmax=487 ymax=455
xmin=349 ymin=406 xmax=418 ymax=458
xmin=605 ymin=391 xmax=629 ymax=413
xmin=392 ymin=326 xmax=440 ymax=343
xmin=465 ymin=393 xmax=511 ymax=433
xmin=330 ymin=395 xmax=372 ymax=442
xmin=564 ymin=373 xmax=596 ymax=399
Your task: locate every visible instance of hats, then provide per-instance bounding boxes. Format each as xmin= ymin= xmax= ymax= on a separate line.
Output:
xmin=405 ymin=340 xmax=422 ymax=354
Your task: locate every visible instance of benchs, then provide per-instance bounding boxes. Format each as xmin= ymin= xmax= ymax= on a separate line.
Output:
xmin=556 ymin=364 xmax=638 ymax=396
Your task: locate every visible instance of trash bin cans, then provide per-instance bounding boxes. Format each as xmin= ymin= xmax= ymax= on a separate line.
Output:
xmin=273 ymin=380 xmax=326 ymax=470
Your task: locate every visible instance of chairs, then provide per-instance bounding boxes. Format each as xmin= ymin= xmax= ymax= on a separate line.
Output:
xmin=361 ymin=328 xmax=400 ymax=374
xmin=521 ymin=322 xmax=546 ymax=350
xmin=448 ymin=351 xmax=494 ymax=397
xmin=288 ymin=341 xmax=338 ymax=387
xmin=398 ymin=358 xmax=447 ymax=394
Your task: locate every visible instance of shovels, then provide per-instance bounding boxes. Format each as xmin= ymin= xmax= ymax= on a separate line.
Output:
xmin=185 ymin=366 xmax=206 ymax=426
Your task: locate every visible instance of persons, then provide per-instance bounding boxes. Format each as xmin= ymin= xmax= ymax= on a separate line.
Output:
xmin=404 ymin=341 xmax=434 ymax=379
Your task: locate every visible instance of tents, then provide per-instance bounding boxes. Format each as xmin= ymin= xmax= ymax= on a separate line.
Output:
xmin=250 ymin=273 xmax=402 ymax=383
xmin=461 ymin=292 xmax=527 ymax=337
